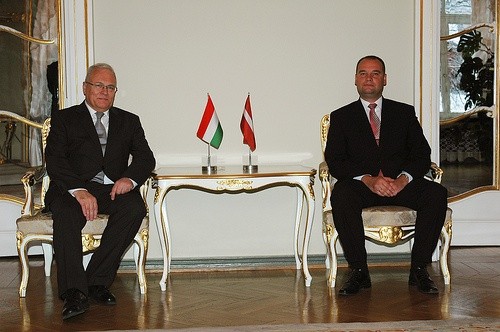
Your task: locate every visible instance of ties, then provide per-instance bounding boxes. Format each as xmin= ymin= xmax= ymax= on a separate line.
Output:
xmin=90 ymin=111 xmax=107 ymax=184
xmin=368 ymin=103 xmax=385 ymax=177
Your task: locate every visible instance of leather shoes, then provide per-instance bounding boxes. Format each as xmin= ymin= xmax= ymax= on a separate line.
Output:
xmin=337 ymin=263 xmax=371 ymax=296
xmin=88 ymin=286 xmax=117 ymax=305
xmin=62 ymin=288 xmax=89 ymax=320
xmin=408 ymin=264 xmax=440 ymax=294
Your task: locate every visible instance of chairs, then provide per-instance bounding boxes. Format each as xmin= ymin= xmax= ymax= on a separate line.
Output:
xmin=319 ymin=115 xmax=453 ymax=287
xmin=17 ymin=117 xmax=151 ymax=297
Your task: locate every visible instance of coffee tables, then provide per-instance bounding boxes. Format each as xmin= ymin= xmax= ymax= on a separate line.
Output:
xmin=152 ymin=163 xmax=318 ymax=292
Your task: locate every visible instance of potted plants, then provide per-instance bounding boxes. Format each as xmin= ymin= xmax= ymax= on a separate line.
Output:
xmin=456 ymin=29 xmax=494 ymax=172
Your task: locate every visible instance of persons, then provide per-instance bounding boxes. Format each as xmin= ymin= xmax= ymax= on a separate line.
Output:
xmin=43 ymin=64 xmax=156 ymax=320
xmin=324 ymin=57 xmax=448 ymax=296
xmin=47 ymin=61 xmax=58 ymax=114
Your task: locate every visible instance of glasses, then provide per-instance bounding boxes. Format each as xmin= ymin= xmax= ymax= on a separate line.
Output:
xmin=85 ymin=82 xmax=115 ymax=91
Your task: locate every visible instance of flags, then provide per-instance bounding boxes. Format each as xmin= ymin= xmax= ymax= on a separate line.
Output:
xmin=196 ymin=96 xmax=223 ymax=149
xmin=239 ymin=95 xmax=257 ymax=152
